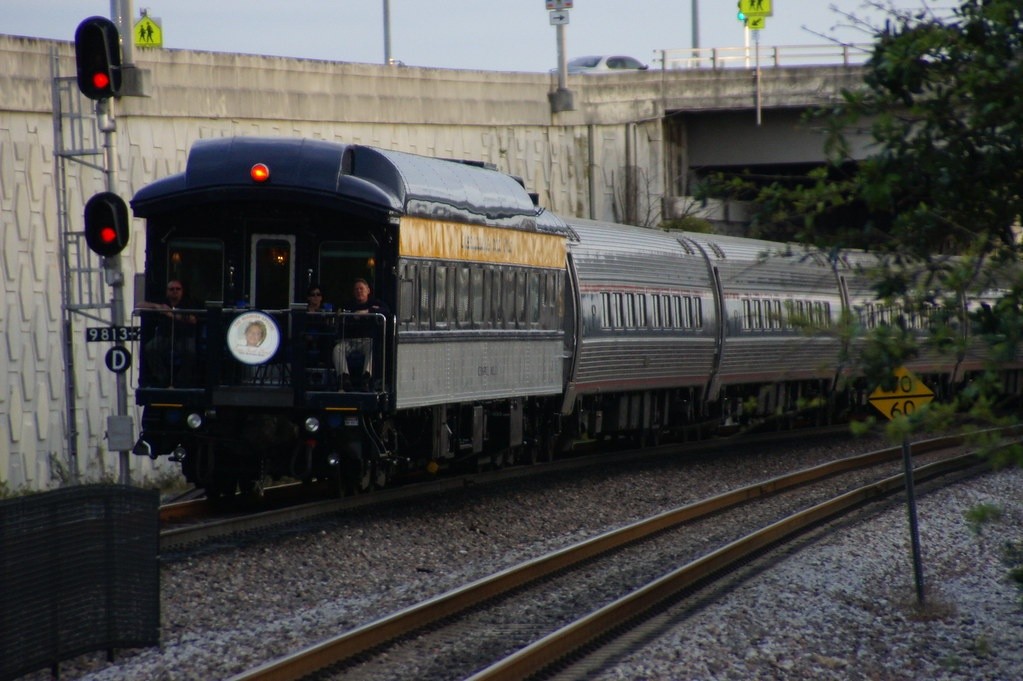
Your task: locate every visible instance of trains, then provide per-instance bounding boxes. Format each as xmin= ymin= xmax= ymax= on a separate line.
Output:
xmin=128 ymin=134 xmax=1022 ymax=518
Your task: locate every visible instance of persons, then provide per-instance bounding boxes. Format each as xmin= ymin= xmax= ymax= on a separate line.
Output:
xmin=297 ymin=286 xmax=334 ymax=384
xmin=332 ymin=279 xmax=390 ymax=391
xmin=136 ymin=280 xmax=202 ymax=387
xmin=246 ymin=321 xmax=267 ymax=346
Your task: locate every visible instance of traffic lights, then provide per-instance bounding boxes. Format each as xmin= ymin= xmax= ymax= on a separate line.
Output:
xmin=74 ymin=15 xmax=124 ymax=102
xmin=82 ymin=191 xmax=130 ymax=260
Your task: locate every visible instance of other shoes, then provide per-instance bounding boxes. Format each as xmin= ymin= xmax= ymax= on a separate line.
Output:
xmin=358 ymin=375 xmax=370 ymax=392
xmin=342 ymin=375 xmax=354 ymax=391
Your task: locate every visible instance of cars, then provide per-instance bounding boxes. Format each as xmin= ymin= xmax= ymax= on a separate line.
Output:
xmin=551 ymin=53 xmax=661 ymax=74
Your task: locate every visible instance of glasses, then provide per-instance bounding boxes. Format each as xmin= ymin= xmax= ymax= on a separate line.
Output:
xmin=167 ymin=287 xmax=182 ymax=292
xmin=307 ymin=292 xmax=321 ymax=297
